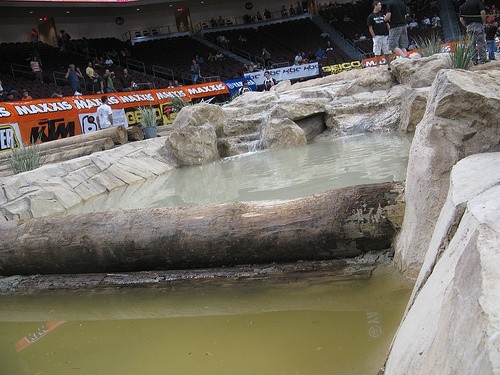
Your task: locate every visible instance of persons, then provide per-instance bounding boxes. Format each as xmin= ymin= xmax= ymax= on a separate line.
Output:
xmin=367 ymin=1 xmax=394 ymax=72
xmin=459 ymin=0 xmax=492 ymax=66
xmin=0 ymin=51 xmax=226 ymax=102
xmin=404 ymin=13 xmax=441 ymax=34
xmin=261 ymin=47 xmax=273 ymax=69
xmin=209 ymin=0 xmax=345 ymax=29
xmin=237 ymin=80 xmax=252 ymax=95
xmin=382 ymin=0 xmax=411 ymax=61
xmin=217 ymin=35 xmax=249 ymax=44
xmin=264 ymin=70 xmax=277 ymax=91
xmin=292 ymin=30 xmax=367 ymax=65
xmin=487 ymin=20 xmax=498 ymax=61
xmin=97 ymin=96 xmax=113 ymax=130
xmin=56 ymin=28 xmax=72 ymax=49
xmin=481 ymin=0 xmax=500 ymax=37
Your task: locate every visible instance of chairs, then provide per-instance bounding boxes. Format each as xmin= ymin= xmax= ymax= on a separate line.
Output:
xmin=0 ymin=0 xmax=500 ymax=104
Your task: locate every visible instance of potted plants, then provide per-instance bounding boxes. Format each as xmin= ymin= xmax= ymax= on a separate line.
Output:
xmin=136 ymin=105 xmax=158 ymax=139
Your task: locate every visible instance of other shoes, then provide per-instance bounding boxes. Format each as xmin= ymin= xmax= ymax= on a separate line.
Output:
xmin=473 ymin=62 xmax=477 ymax=65
xmin=480 ymin=59 xmax=490 ymax=63
